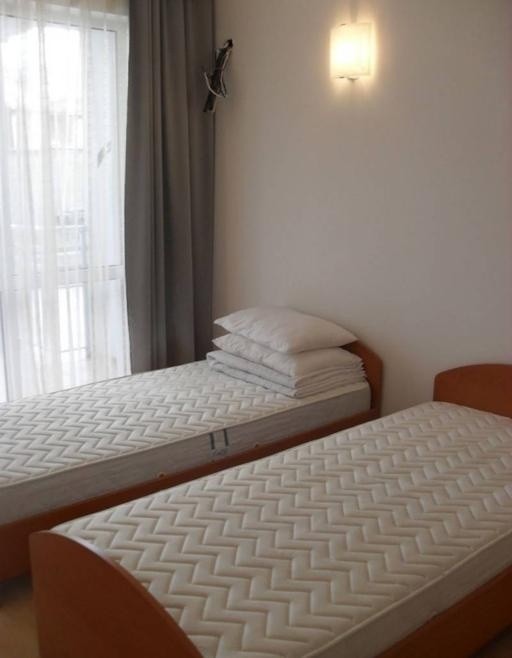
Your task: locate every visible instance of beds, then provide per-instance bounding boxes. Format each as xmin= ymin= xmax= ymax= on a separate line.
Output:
xmin=0 ymin=342 xmax=383 ymax=581
xmin=30 ymin=362 xmax=511 ymax=657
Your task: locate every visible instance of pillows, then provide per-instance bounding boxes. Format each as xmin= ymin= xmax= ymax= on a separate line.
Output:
xmin=210 ymin=307 xmax=364 ymax=377
